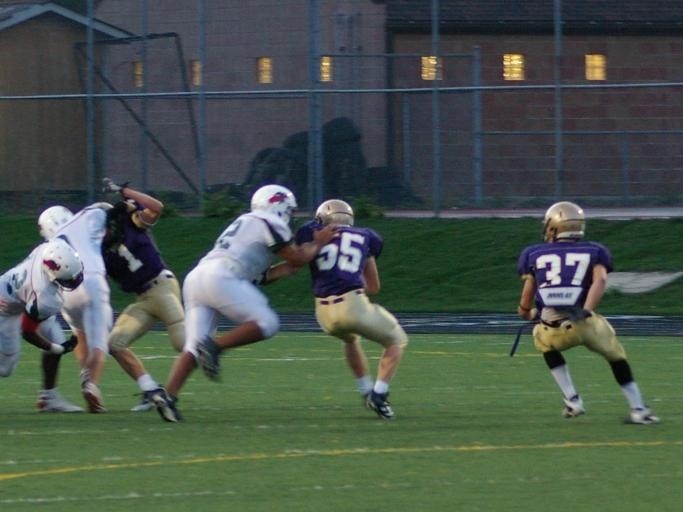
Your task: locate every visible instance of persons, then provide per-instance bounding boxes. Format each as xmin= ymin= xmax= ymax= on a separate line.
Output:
xmin=143 ymin=182 xmax=342 ymax=424
xmin=37 ymin=200 xmax=115 ymax=414
xmin=96 ymin=177 xmax=187 ymax=413
xmin=252 ymin=198 xmax=409 ymax=420
xmin=517 ymin=200 xmax=661 ymax=426
xmin=0 ymin=237 xmax=84 ymax=414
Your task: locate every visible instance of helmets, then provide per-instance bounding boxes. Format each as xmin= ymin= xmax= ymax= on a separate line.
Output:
xmin=542 ymin=201 xmax=586 ymax=243
xmin=314 ymin=199 xmax=355 ymax=226
xmin=41 ymin=238 xmax=84 ymax=292
xmin=37 ymin=205 xmax=74 ymax=242
xmin=250 ymin=185 xmax=298 ymax=225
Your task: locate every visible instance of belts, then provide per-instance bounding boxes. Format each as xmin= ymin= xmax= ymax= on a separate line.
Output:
xmin=320 ymin=290 xmax=361 ymax=305
xmin=136 ymin=275 xmax=173 ymax=295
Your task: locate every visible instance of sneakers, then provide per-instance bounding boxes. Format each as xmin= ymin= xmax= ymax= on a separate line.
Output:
xmin=38 ymin=336 xmax=222 ymax=425
xmin=560 ymin=393 xmax=585 ymax=417
xmin=629 ymin=404 xmax=660 ymax=424
xmin=362 ymin=390 xmax=396 ymax=421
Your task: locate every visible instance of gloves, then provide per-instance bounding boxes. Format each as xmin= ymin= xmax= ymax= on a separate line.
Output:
xmin=102 ymin=178 xmax=123 ymax=194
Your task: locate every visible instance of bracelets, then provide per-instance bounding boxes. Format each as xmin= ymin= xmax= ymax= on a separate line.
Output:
xmin=49 ymin=343 xmax=65 ymax=356
